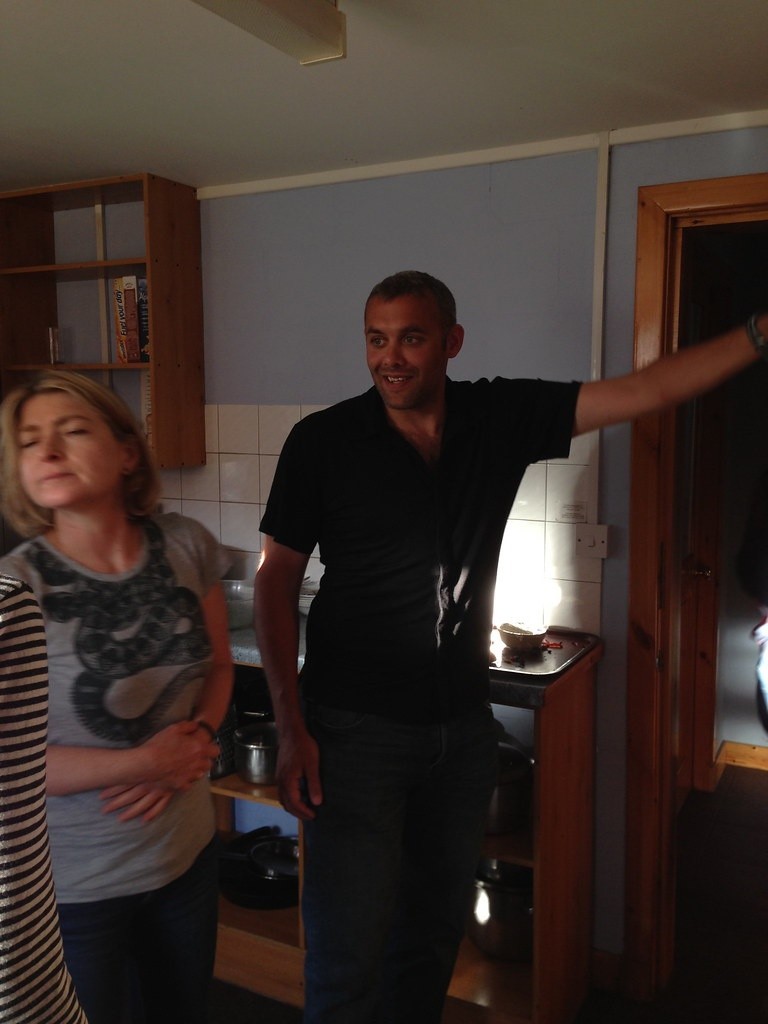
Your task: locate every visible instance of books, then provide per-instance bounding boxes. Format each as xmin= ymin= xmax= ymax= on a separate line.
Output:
xmin=111 ymin=277 xmax=158 ymax=451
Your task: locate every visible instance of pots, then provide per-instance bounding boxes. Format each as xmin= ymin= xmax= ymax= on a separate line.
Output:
xmin=216 ymin=841 xmax=302 ymax=877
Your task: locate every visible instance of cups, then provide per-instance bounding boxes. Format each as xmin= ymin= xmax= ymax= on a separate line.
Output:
xmin=42 ymin=327 xmax=65 ymax=364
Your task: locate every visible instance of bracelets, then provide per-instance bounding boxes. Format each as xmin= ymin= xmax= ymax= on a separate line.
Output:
xmin=747 ymin=312 xmax=768 ymax=355
xmin=197 ymin=721 xmax=219 ymax=744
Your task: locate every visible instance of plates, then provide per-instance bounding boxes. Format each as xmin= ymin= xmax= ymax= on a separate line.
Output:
xmin=299 ymin=595 xmax=315 ymax=616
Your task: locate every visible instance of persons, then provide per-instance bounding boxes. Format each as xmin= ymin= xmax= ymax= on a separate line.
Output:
xmin=252 ymin=269 xmax=768 ymax=1024
xmin=0 ymin=364 xmax=235 ymax=1023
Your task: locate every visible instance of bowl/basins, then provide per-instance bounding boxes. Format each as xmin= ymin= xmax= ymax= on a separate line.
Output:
xmin=235 ymin=723 xmax=279 ymax=784
xmin=498 ymin=624 xmax=547 ymax=650
xmin=221 ymin=580 xmax=254 ymax=630
xmin=469 ymin=861 xmax=531 ymax=962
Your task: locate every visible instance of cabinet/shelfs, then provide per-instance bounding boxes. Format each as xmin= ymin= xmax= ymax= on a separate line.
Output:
xmin=210 ymin=647 xmax=600 ymax=1024
xmin=0 ymin=173 xmax=206 ymax=472
